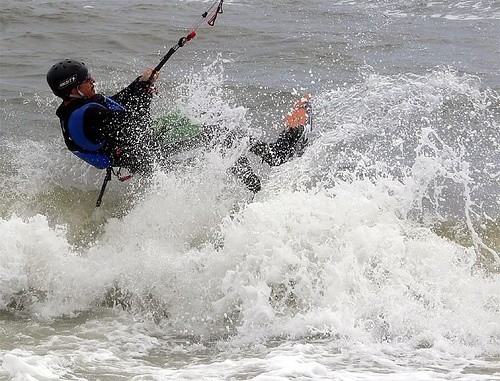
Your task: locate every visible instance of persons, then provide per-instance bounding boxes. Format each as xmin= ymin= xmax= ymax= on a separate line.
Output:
xmin=45 ymin=58 xmax=305 ymax=200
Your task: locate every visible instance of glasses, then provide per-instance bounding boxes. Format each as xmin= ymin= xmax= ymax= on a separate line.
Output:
xmin=81 ymin=73 xmax=92 ymax=84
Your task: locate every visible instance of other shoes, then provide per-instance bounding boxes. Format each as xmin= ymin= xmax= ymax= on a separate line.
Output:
xmin=262 ymin=123 xmax=306 ymax=166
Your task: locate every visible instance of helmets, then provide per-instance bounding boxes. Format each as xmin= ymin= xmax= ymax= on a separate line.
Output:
xmin=46 ymin=59 xmax=88 ymax=99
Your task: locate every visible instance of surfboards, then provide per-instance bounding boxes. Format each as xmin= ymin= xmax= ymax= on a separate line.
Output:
xmin=288 ymin=94 xmax=311 ymax=160
